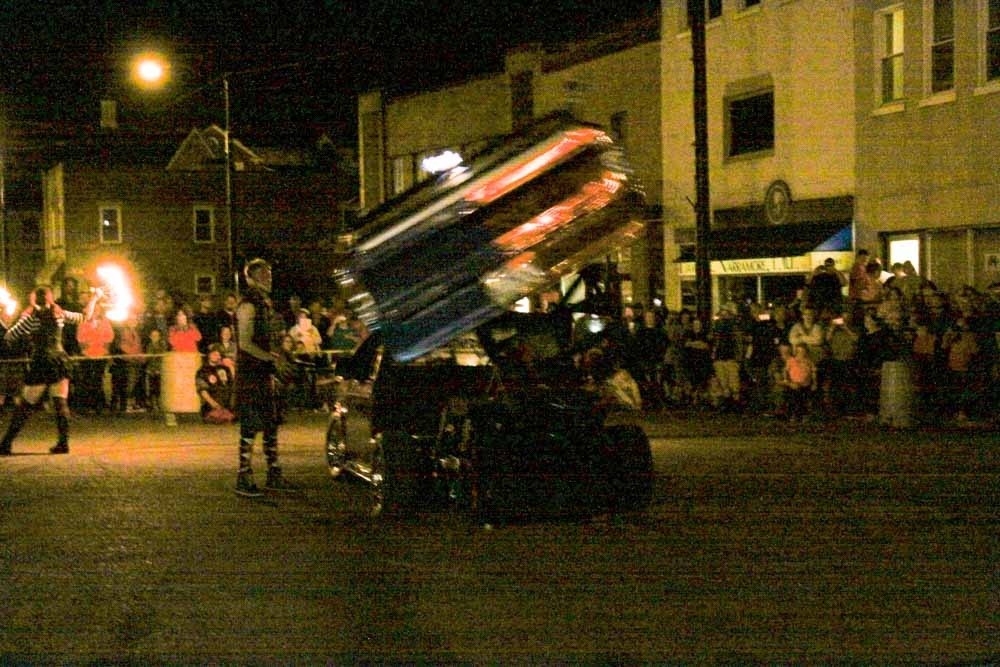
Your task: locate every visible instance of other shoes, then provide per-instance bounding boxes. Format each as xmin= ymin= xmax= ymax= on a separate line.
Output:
xmin=236 ymin=468 xmax=264 ymax=497
xmin=265 ymin=468 xmax=298 ymax=493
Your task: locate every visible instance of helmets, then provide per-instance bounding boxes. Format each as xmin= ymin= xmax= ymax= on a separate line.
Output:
xmin=280 ymin=350 xmax=316 ymax=384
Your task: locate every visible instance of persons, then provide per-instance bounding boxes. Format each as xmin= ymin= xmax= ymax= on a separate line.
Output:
xmin=232 ymin=258 xmax=300 ymax=497
xmin=0 ymin=287 xmax=88 ymax=455
xmin=0 ymin=276 xmax=367 ymax=427
xmin=574 ymin=249 xmax=999 ymax=421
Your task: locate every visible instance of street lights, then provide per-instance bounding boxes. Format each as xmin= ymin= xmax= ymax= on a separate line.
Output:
xmin=131 ymin=50 xmax=237 ymax=290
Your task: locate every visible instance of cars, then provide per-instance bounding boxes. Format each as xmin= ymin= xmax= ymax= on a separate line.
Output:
xmin=323 ymin=309 xmax=655 ymax=526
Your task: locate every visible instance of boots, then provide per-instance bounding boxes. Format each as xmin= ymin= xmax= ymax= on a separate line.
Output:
xmin=51 ymin=396 xmax=70 ymax=452
xmin=1 ymin=398 xmax=40 ymax=457
